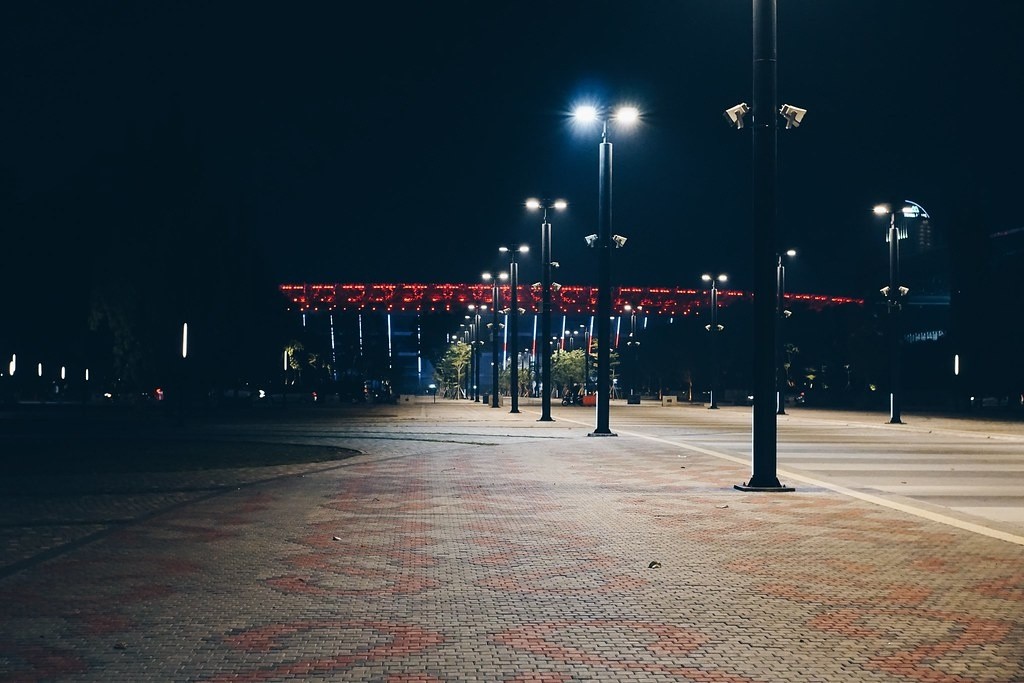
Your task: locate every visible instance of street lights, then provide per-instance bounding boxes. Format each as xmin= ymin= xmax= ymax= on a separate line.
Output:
xmin=873 ymin=204 xmax=918 ymax=423
xmin=482 ymin=273 xmax=508 ymax=407
xmin=497 ymin=245 xmax=531 ymax=413
xmin=702 ymin=273 xmax=728 ymax=411
xmin=568 ymin=100 xmax=635 ymax=438
xmin=523 ymin=196 xmax=568 ymax=422
xmin=774 ymin=246 xmax=798 ymax=413
xmin=467 ymin=303 xmax=488 ymax=406
